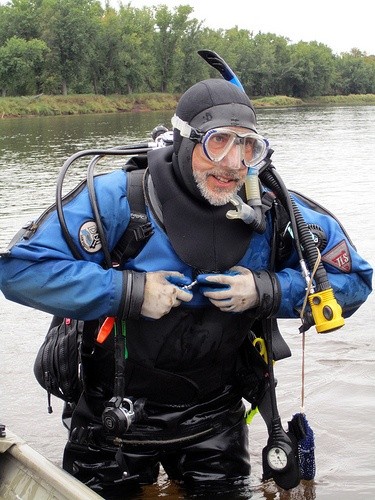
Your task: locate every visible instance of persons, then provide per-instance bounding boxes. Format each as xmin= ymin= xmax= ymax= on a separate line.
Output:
xmin=0 ymin=80 xmax=373 ymax=500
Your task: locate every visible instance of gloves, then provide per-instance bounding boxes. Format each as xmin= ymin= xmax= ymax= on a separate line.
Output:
xmin=197 ymin=266 xmax=282 ymax=320
xmin=117 ymin=270 xmax=193 ymax=322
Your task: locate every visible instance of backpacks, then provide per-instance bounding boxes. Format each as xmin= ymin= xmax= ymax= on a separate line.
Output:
xmin=34 ymin=154 xmax=286 ymax=413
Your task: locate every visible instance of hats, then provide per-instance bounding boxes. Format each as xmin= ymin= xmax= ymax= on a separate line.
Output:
xmin=147 ymin=78 xmax=266 ymax=274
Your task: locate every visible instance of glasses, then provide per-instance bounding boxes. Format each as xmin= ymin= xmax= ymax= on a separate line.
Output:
xmin=202 ymin=128 xmax=270 ymax=170
